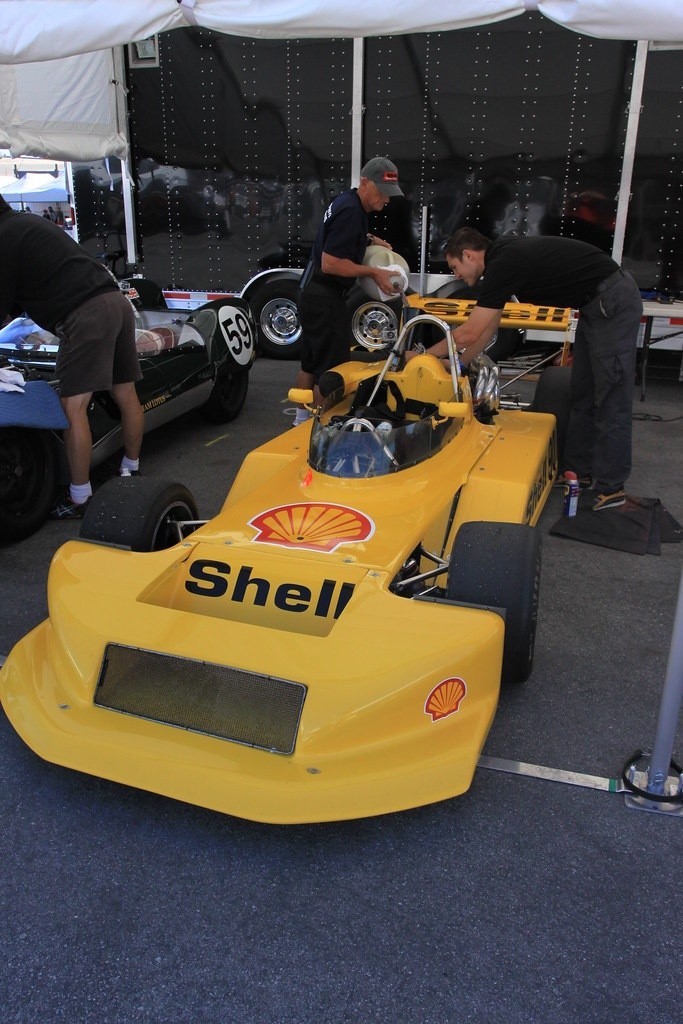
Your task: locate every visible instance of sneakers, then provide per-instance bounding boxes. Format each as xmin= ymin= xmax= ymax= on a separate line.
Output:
xmin=552 ymin=470 xmax=595 ymax=490
xmin=45 ymin=489 xmax=94 ymax=520
xmin=577 ymin=485 xmax=626 ymax=511
xmin=109 ymin=463 xmax=140 ymax=481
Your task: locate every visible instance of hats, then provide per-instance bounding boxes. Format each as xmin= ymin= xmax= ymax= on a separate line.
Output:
xmin=361 ymin=156 xmax=405 ymax=197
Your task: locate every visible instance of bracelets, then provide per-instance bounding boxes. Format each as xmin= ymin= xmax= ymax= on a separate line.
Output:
xmin=460 ymin=360 xmax=467 ymax=371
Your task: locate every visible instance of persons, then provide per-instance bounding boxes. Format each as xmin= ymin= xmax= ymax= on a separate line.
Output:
xmin=42 ymin=206 xmax=65 ymax=225
xmin=25 ymin=206 xmax=32 ymax=213
xmin=404 ymin=228 xmax=643 ymax=511
xmin=290 ymin=156 xmax=406 ymax=428
xmin=0 ymin=195 xmax=144 ymax=520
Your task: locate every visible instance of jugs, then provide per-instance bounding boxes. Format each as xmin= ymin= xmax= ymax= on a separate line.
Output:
xmin=354 ymin=245 xmax=410 ymax=302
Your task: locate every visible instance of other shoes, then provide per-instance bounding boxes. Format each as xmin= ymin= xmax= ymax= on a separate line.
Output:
xmin=292 ymin=418 xmax=308 ymax=426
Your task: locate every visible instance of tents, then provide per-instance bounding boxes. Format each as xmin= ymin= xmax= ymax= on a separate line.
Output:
xmin=1 ymin=172 xmax=73 ymax=211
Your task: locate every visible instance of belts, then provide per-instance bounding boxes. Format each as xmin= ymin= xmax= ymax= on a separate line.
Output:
xmin=579 ymin=269 xmax=625 ymax=307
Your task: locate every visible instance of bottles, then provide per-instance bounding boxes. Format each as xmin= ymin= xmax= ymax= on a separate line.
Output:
xmin=562 ymin=471 xmax=580 ymax=518
